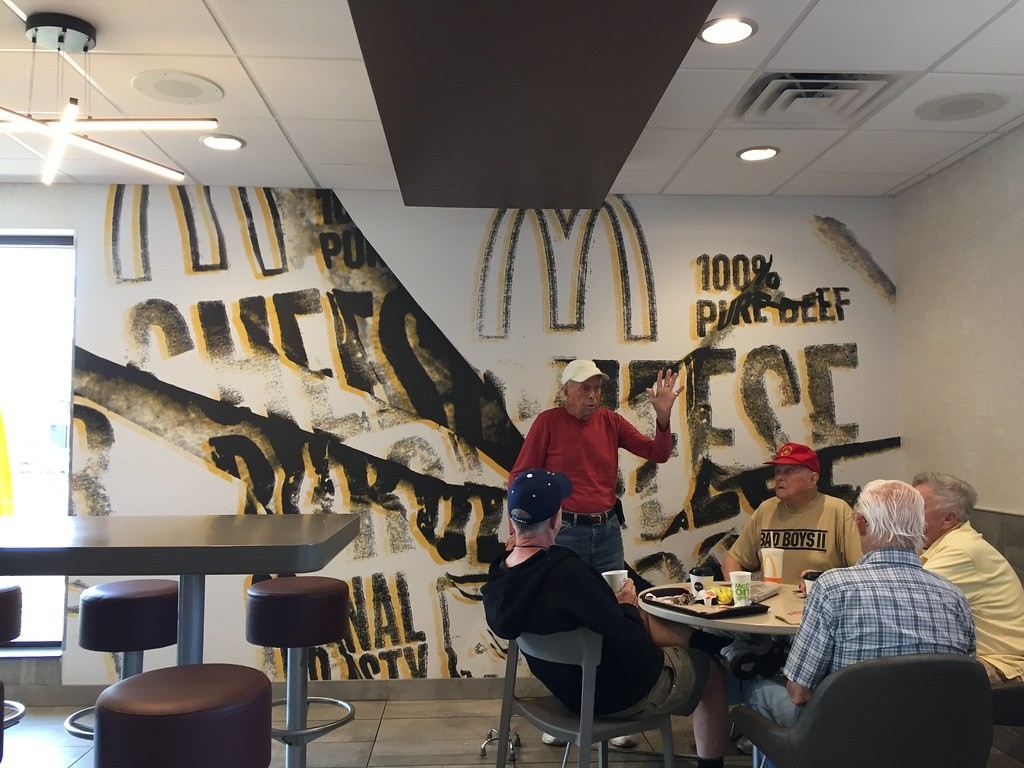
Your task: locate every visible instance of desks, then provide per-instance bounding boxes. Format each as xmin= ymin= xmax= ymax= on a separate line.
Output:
xmin=0 ymin=513 xmax=360 ymax=768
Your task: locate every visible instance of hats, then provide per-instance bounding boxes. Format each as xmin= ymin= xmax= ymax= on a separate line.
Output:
xmin=507 ymin=468 xmax=573 ymax=525
xmin=561 ymin=360 xmax=609 ymax=386
xmin=762 ymin=442 xmax=820 ymax=474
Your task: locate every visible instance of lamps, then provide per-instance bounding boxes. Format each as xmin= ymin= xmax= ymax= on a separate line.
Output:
xmin=0 ymin=12 xmax=220 ymax=186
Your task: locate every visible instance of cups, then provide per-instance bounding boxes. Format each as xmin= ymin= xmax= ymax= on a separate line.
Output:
xmin=802 ymin=572 xmax=823 ymax=596
xmin=689 ymin=567 xmax=715 ymax=599
xmin=761 ymin=548 xmax=784 ymax=584
xmin=602 ymin=570 xmax=628 ymax=593
xmin=729 ymin=571 xmax=752 ymax=606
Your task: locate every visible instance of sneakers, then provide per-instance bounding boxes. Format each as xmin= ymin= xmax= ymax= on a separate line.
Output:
xmin=720 ymin=639 xmax=785 ymax=681
xmin=541 ymin=731 xmax=570 ymax=747
xmin=611 ymin=736 xmax=639 ymax=748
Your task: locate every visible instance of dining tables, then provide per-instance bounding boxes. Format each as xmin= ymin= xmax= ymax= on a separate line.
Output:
xmin=638 ymin=580 xmax=809 ymax=768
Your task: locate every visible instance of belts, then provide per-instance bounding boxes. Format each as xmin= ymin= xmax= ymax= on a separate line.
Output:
xmin=562 ymin=506 xmax=616 ymax=526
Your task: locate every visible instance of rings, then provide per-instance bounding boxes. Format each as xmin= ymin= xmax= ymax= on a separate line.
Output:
xmin=664 ymin=383 xmax=669 ymax=387
xmin=674 ymin=392 xmax=679 ymax=396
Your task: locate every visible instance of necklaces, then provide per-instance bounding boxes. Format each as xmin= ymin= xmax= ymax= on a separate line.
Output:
xmin=512 ymin=544 xmax=549 ymax=550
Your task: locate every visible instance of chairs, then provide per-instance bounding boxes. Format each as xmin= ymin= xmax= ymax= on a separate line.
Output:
xmin=730 ymin=653 xmax=994 ymax=768
xmin=496 ymin=627 xmax=674 ymax=768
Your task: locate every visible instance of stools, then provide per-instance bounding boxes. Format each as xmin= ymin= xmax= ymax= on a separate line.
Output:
xmin=245 ymin=576 xmax=355 ymax=744
xmin=0 ymin=585 xmax=26 ymax=727
xmin=93 ymin=663 xmax=272 ymax=768
xmin=63 ymin=579 xmax=178 ymax=740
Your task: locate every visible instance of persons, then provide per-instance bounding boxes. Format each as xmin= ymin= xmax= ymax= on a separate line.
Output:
xmin=743 ymin=479 xmax=977 ymax=768
xmin=480 ymin=468 xmax=772 ymax=768
xmin=912 ymin=472 xmax=1024 ymax=688
xmin=721 ymin=443 xmax=863 ymax=755
xmin=505 ymin=360 xmax=685 ymax=747
